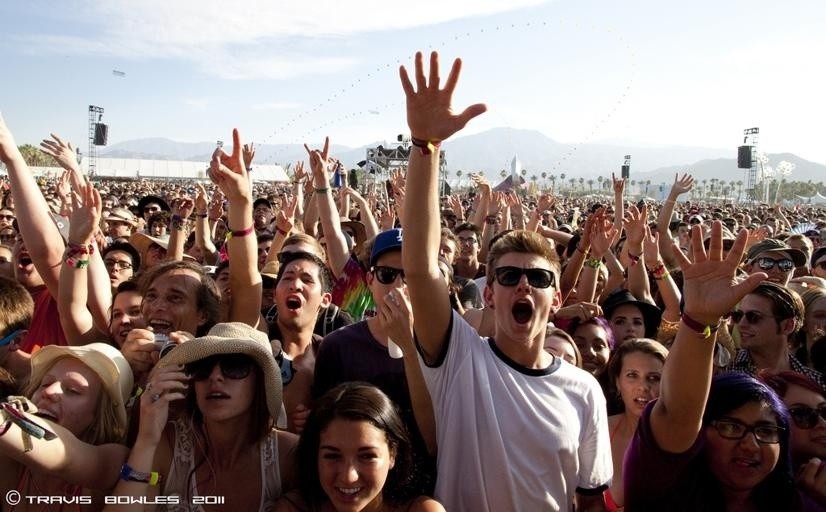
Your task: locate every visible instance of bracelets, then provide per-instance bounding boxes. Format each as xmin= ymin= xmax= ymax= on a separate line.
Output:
xmin=410 ymin=132 xmax=446 ymax=155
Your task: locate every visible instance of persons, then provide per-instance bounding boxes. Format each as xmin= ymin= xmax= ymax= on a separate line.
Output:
xmin=396 ymin=50 xmax=615 ymax=512
xmin=2 ymin=126 xmax=826 ymax=510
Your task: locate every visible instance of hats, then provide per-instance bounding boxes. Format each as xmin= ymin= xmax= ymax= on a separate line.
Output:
xmin=602 ymin=289 xmax=661 ymax=322
xmin=129 ymin=232 xmax=196 ymax=261
xmin=370 ymin=227 xmax=402 ymax=265
xmin=105 ymin=208 xmax=137 ymax=227
xmin=30 ymin=342 xmax=135 ymax=441
xmin=704 ymin=225 xmax=736 ymax=242
xmin=138 ymin=195 xmax=171 ymax=218
xmin=317 ymin=217 xmax=368 ymax=243
xmin=13 ymin=209 xmax=69 ymax=246
xmin=558 ymin=224 xmax=572 ymax=231
xmin=156 ymin=321 xmax=288 ymax=429
xmin=260 ymin=261 xmax=280 ymax=280
xmin=747 ymin=239 xmax=806 ymax=267
xmin=688 ymin=214 xmax=704 ymax=224
xmin=253 ymin=198 xmax=271 ymax=209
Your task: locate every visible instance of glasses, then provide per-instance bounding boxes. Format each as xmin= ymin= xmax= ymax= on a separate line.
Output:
xmin=459 ymin=238 xmax=478 ymax=245
xmin=752 ymin=257 xmax=795 ymax=272
xmin=730 ymin=311 xmax=763 ymax=325
xmin=814 ymin=261 xmax=826 ymax=271
xmin=104 ymin=258 xmax=133 ymax=269
xmin=0 ymin=214 xmax=16 ymax=221
xmin=374 ymin=265 xmax=408 ymax=285
xmin=787 ymin=407 xmax=815 ymax=430
xmin=709 ymin=419 xmax=787 ymax=444
xmin=705 ymin=242 xmax=732 ymax=251
xmin=143 ymin=207 xmax=159 ymax=214
xmin=492 ymin=265 xmax=557 ymax=289
xmin=255 ymin=207 xmax=271 ymax=213
xmin=190 ymin=354 xmax=253 ymax=381
xmin=277 ymin=252 xmax=292 ymax=263
xmin=346 ymin=231 xmax=356 ymax=238
